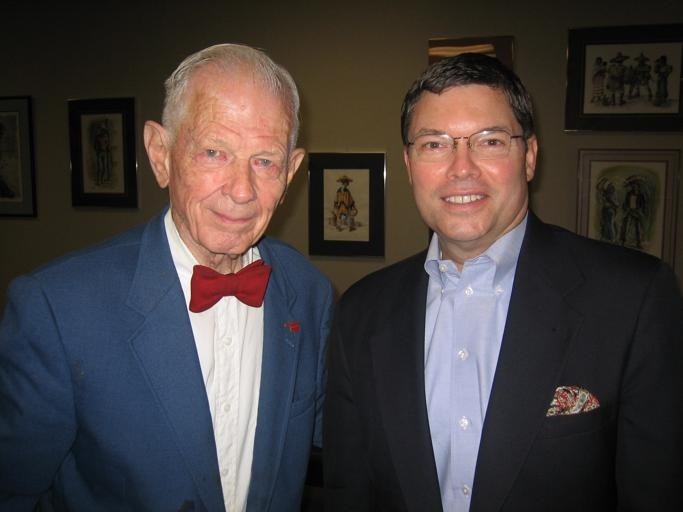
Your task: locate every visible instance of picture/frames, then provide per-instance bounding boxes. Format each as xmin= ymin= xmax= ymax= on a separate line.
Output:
xmin=564 ymin=22 xmax=683 ymax=132
xmin=68 ymin=95 xmax=138 ymax=209
xmin=308 ymin=152 xmax=387 ymax=257
xmin=0 ymin=95 xmax=39 ymax=219
xmin=575 ymin=147 xmax=682 ymax=274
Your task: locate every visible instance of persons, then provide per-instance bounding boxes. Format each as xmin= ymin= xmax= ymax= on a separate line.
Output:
xmin=588 ymin=57 xmax=607 ymax=104
xmin=319 ymin=48 xmax=683 ymax=512
xmin=607 ymin=52 xmax=629 ymax=107
xmin=600 ymin=182 xmax=619 ymax=241
xmin=652 ymin=55 xmax=674 ymax=107
xmin=632 ymin=52 xmax=653 ymax=103
xmin=620 ymin=183 xmax=646 ymax=250
xmin=0 ymin=40 xmax=336 ymax=510
xmin=332 ymin=175 xmax=358 ymax=233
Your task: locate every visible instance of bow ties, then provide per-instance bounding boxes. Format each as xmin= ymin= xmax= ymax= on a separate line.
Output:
xmin=189 ymin=258 xmax=272 ymax=313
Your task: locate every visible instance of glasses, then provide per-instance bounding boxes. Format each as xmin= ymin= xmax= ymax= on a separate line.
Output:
xmin=406 ymin=129 xmax=525 ymax=164
